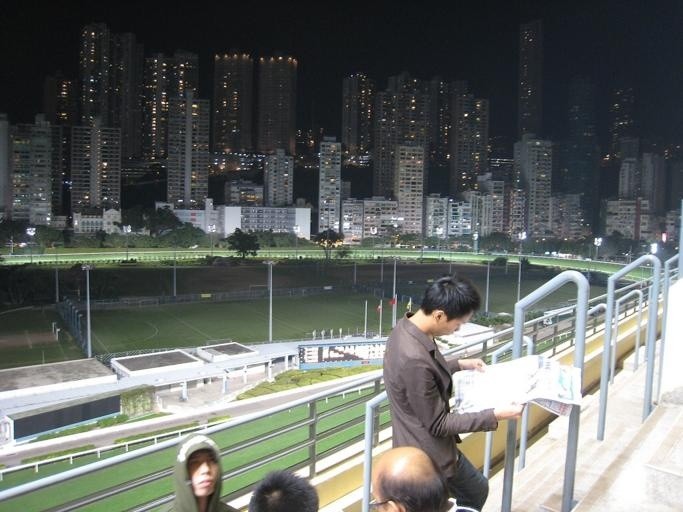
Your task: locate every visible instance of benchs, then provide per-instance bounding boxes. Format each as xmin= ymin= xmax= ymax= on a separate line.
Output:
xmin=311 ymin=301 xmax=662 ymax=511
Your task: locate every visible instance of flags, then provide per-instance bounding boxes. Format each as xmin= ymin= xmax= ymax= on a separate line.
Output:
xmin=377 ymin=305 xmax=381 ymax=312
xmin=389 ymin=295 xmax=395 ymax=305
xmin=406 ymin=299 xmax=410 ymax=310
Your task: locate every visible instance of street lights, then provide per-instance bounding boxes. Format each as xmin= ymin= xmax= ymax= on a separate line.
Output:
xmin=436 ymin=225 xmax=443 ymax=259
xmin=207 ymin=223 xmax=216 ymax=257
xmin=594 ymin=237 xmax=602 ymax=258
xmin=518 ymin=231 xmax=526 ymax=253
xmin=293 ymin=225 xmax=301 ymax=258
xmin=27 ymin=227 xmax=36 ymax=262
xmin=123 ymin=224 xmax=132 ymax=259
xmin=262 ymin=260 xmax=279 ymax=344
xmin=370 ymin=226 xmax=377 ymax=257
xmin=80 ymin=263 xmax=97 ymax=359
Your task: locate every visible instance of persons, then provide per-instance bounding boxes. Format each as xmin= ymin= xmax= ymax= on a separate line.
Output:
xmin=382 ymin=275 xmax=527 ymax=512
xmin=162 ymin=433 xmax=240 ymax=511
xmin=247 ymin=468 xmax=318 ymax=512
xmin=368 ymin=445 xmax=482 ymax=512
